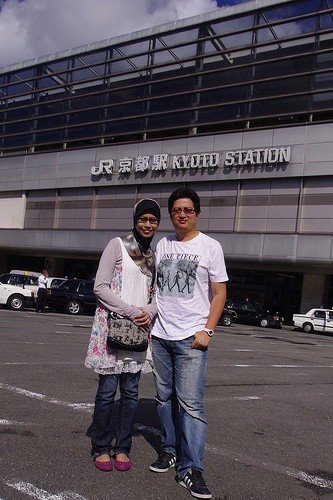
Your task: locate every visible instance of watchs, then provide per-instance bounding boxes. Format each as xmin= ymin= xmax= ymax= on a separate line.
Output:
xmin=202 ymin=327 xmax=214 ymax=337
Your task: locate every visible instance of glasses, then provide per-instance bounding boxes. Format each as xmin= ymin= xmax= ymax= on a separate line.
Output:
xmin=170 ymin=206 xmax=198 ymax=213
xmin=137 ymin=217 xmax=159 ymax=224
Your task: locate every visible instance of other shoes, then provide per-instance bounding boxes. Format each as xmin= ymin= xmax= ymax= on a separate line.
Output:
xmin=94 ymin=454 xmax=113 ymax=472
xmin=114 ymin=453 xmax=132 ymax=471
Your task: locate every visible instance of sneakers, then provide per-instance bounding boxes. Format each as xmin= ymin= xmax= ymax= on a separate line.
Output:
xmin=149 ymin=452 xmax=178 ymax=472
xmin=177 ymin=467 xmax=212 ymax=499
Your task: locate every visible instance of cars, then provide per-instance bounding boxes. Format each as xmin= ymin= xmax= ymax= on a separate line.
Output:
xmin=293 ymin=308 xmax=333 ymax=334
xmin=224 ymin=298 xmax=281 ymax=328
xmin=36 ymin=279 xmax=97 ymax=315
xmin=0 ymin=269 xmax=68 ymax=311
xmin=217 ymin=308 xmax=238 ymax=326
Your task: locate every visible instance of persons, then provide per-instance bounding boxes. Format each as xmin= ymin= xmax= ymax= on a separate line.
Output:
xmin=35 ymin=268 xmax=50 ymax=313
xmin=139 ymin=188 xmax=231 ymax=498
xmin=84 ymin=197 xmax=160 ymax=473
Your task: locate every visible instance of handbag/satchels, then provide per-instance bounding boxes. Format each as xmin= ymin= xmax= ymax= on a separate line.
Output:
xmin=106 ymin=311 xmax=148 ymax=352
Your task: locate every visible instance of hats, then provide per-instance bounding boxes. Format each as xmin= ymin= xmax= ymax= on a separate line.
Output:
xmin=131 ymin=197 xmax=162 ymax=224
